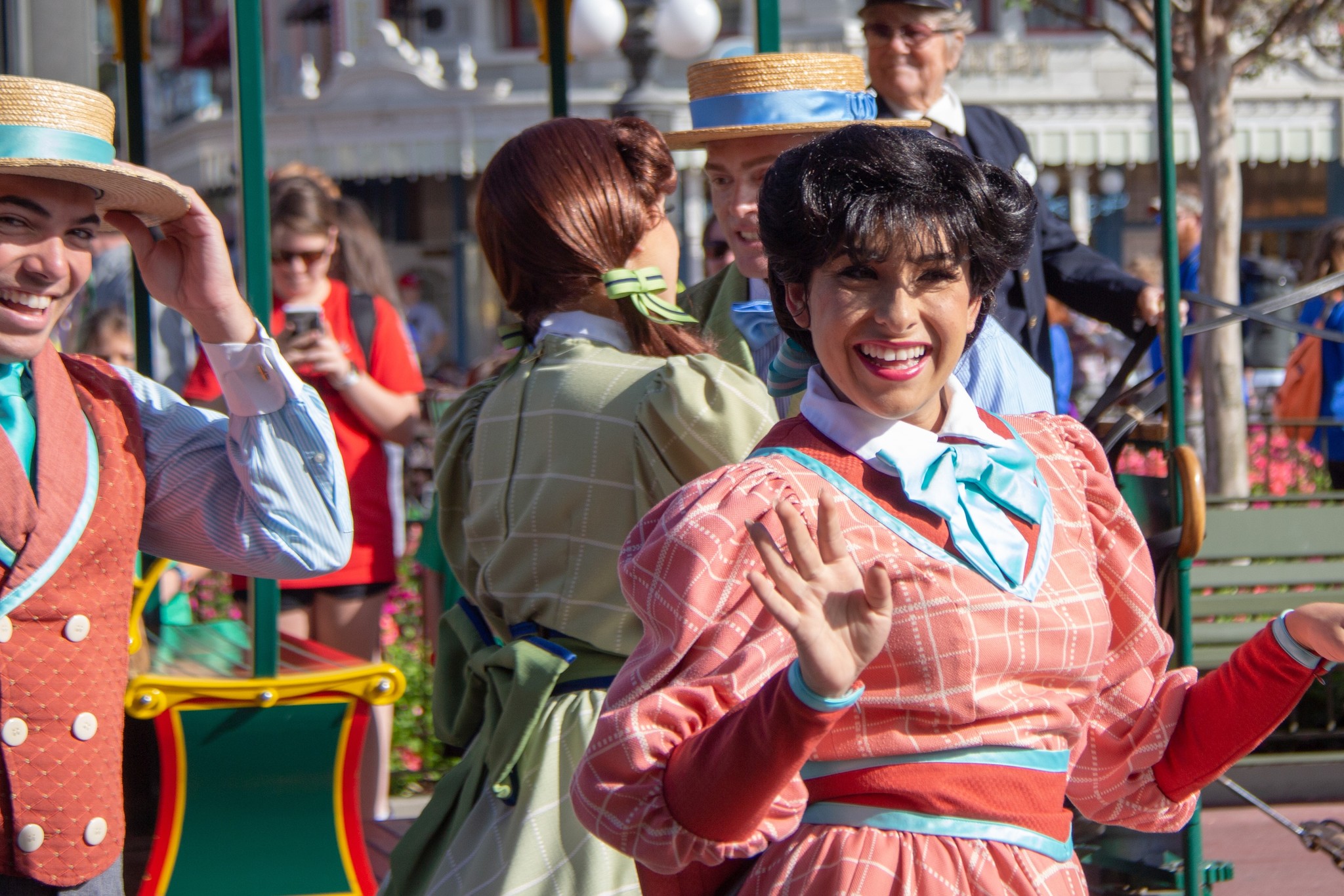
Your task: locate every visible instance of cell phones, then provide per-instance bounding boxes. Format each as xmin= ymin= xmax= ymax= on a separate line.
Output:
xmin=287 ymin=304 xmax=319 ymax=352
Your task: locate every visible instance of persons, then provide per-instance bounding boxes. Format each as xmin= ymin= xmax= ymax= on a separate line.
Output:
xmin=0 ymin=74 xmax=351 ymax=895
xmin=69 ymin=214 xmax=217 ymax=626
xmin=373 ymin=115 xmax=783 ymax=896
xmin=1295 ymin=240 xmax=1344 ymax=506
xmin=179 ymin=161 xmax=425 ymax=820
xmin=659 ymin=53 xmax=1056 ymax=420
xmin=857 ymin=1 xmax=1190 ymax=417
xmin=1149 ymin=193 xmax=1213 ymax=475
xmin=568 ymin=126 xmax=1344 ymax=896
xmin=270 ymin=164 xmax=419 ymax=557
xmin=415 ymin=491 xmax=475 ymax=667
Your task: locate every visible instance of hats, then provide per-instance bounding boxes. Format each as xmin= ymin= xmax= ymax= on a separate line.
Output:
xmin=857 ymin=0 xmax=963 ymax=25
xmin=0 ymin=71 xmax=191 ymax=232
xmin=659 ymin=53 xmax=933 ymax=151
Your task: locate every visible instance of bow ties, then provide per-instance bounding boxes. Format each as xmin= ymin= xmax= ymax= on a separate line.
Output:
xmin=876 ymin=440 xmax=1052 ymax=593
xmin=729 ymin=300 xmax=782 ymax=351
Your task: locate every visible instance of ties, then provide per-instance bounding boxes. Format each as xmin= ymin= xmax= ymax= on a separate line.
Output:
xmin=0 ymin=361 xmax=37 ymax=482
xmin=922 ymin=116 xmax=949 ymax=140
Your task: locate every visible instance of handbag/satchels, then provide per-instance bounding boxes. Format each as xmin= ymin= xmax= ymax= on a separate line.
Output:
xmin=1275 ymin=316 xmax=1326 ymax=441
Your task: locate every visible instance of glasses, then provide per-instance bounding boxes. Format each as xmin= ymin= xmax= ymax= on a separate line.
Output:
xmin=273 ymin=244 xmax=327 ymax=268
xmin=862 ymin=21 xmax=959 ymax=54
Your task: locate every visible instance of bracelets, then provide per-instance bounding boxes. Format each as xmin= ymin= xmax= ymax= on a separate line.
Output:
xmin=330 ymin=362 xmax=360 ymax=392
